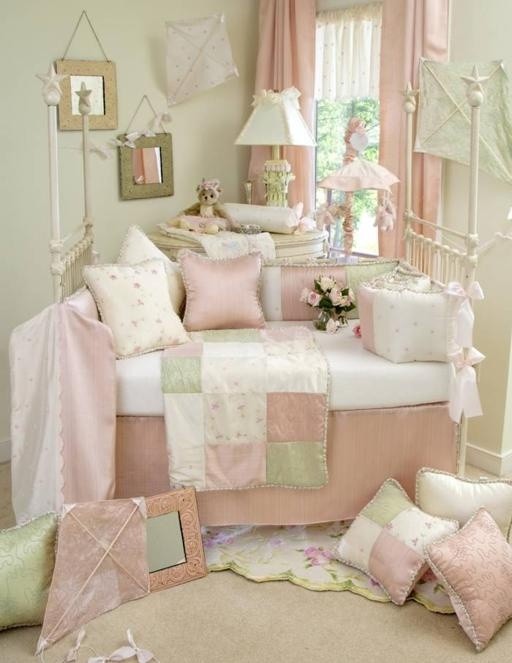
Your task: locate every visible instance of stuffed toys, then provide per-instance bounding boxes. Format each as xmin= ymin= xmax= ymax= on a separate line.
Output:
xmin=166 ymin=176 xmax=242 ymax=235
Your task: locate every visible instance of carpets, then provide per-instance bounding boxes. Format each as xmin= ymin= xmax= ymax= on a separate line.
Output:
xmin=200 ymin=524 xmax=468 ymax=614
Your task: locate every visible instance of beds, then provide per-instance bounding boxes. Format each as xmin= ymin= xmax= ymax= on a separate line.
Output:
xmin=37 ymin=64 xmax=490 ymax=529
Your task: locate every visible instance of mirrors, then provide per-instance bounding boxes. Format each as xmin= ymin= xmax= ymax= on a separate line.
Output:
xmin=55 ymin=59 xmax=118 ymax=131
xmin=118 ymin=132 xmax=174 ymax=200
xmin=136 ymin=487 xmax=207 ymax=597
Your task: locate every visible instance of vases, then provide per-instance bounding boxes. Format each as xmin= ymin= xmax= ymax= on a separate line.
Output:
xmin=335 ymin=312 xmax=349 ymax=327
xmin=312 ymin=310 xmax=333 ymax=331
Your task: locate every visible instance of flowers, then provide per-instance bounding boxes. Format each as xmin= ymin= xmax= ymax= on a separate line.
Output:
xmin=337 ymin=287 xmax=355 ymax=310
xmin=299 ymin=275 xmax=343 ymax=309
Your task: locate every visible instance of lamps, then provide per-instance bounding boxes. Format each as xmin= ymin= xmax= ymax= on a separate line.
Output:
xmin=234 ymin=89 xmax=318 ymax=207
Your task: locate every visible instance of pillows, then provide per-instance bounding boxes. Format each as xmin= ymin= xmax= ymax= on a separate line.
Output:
xmin=82 ymin=260 xmax=192 ymax=360
xmin=115 ymin=224 xmax=184 ymax=312
xmin=421 ymin=508 xmax=512 ymax=652
xmin=414 ymin=467 xmax=512 ymax=542
xmin=178 ymin=248 xmax=267 ymax=330
xmin=0 ymin=511 xmax=61 ymax=632
xmin=331 ymin=477 xmax=460 ymax=605
xmin=225 ymin=201 xmax=303 ymax=234
